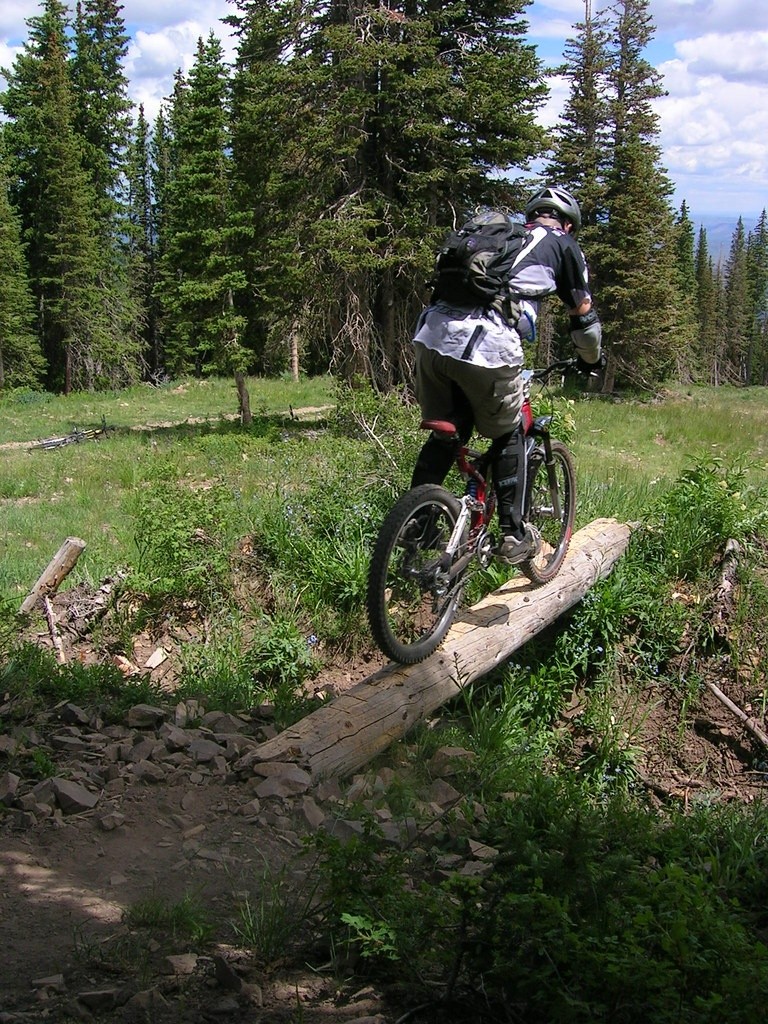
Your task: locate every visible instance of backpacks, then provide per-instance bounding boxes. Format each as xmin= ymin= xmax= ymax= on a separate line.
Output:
xmin=428 ymin=211 xmax=547 ymax=327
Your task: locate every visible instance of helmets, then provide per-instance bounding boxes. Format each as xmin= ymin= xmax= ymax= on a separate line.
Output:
xmin=525 ymin=186 xmax=581 ymax=241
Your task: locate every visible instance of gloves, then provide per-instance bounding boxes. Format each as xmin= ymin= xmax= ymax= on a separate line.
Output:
xmin=574 ymin=355 xmax=604 ymax=376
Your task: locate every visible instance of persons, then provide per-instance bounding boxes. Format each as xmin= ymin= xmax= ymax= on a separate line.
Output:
xmin=404 ymin=187 xmax=609 ymax=564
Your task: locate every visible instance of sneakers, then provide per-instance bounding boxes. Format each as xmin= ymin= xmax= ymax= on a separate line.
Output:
xmin=496 ymin=519 xmax=542 ymax=564
xmin=396 ymin=516 xmax=443 ymax=550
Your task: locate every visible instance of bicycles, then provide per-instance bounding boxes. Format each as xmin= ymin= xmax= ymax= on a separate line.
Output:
xmin=367 ymin=358 xmax=606 ymax=665
